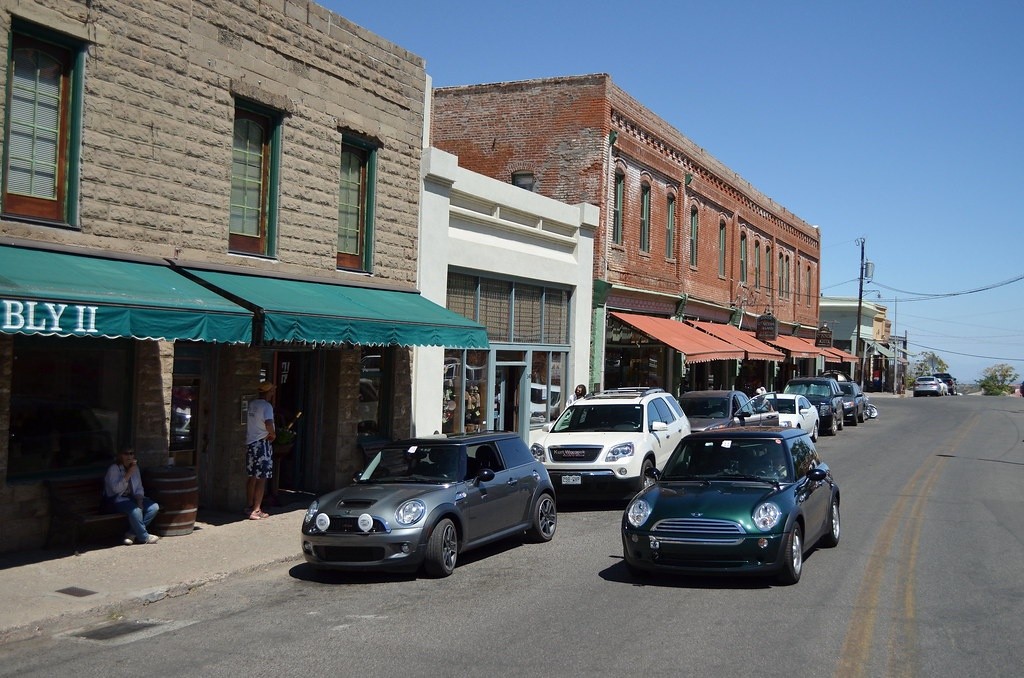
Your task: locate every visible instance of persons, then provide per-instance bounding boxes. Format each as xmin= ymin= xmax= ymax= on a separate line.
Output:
xmin=103 ymin=443 xmax=160 ymax=544
xmin=243 ymin=382 xmax=276 ymax=520
xmin=565 ymin=384 xmax=587 ymax=423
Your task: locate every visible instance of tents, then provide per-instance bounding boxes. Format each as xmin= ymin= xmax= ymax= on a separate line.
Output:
xmin=609 ymin=312 xmax=819 ymax=365
xmin=799 ymin=337 xmax=859 ymax=363
xmin=862 ymin=339 xmax=917 ymax=365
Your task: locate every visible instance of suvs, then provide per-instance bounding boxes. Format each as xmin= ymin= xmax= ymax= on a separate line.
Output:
xmin=932 ymin=372 xmax=958 ymax=396
xmin=531 ymin=387 xmax=691 ymax=500
xmin=780 ymin=377 xmax=844 ymax=436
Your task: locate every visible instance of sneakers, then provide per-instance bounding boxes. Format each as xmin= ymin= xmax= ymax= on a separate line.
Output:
xmin=123 ymin=531 xmax=137 ymax=545
xmin=144 ymin=534 xmax=159 ymax=544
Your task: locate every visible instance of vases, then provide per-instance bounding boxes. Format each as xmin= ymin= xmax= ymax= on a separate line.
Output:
xmin=277 ymin=445 xmax=289 ymax=453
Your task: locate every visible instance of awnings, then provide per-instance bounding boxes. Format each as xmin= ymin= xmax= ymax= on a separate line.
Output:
xmin=0 ymin=245 xmax=254 ymax=345
xmin=182 ymin=267 xmax=490 ymax=349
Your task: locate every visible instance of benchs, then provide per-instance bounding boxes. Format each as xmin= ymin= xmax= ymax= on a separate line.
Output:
xmin=41 ymin=477 xmax=169 ymax=555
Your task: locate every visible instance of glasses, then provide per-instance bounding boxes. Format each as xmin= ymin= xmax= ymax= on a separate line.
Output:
xmin=124 ymin=452 xmax=135 ymax=456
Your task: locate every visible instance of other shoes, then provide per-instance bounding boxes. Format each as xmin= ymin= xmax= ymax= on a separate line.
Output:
xmin=244 ymin=507 xmax=270 ymax=520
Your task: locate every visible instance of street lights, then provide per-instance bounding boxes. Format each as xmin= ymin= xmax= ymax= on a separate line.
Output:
xmin=854 ymin=289 xmax=881 ymax=383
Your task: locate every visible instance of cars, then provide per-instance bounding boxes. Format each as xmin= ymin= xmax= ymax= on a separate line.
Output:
xmin=620 ymin=426 xmax=841 ymax=585
xmin=676 ymin=390 xmax=778 ymax=433
xmin=818 ymin=381 xmax=866 ymax=426
xmin=751 ymin=393 xmax=820 ymax=442
xmin=301 ymin=429 xmax=558 ymax=578
xmin=913 ymin=375 xmax=948 ymax=397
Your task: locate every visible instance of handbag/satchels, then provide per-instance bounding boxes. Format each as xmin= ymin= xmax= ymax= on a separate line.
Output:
xmin=97 ymin=493 xmax=118 ymax=515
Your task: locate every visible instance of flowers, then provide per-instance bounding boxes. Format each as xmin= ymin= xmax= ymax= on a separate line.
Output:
xmin=276 ymin=426 xmax=297 ymax=446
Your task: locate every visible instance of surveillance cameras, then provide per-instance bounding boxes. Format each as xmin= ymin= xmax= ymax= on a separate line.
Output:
xmin=878 ymin=293 xmax=881 ymax=298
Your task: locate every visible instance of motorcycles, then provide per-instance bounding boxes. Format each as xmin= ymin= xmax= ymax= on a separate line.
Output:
xmin=862 ymin=396 xmax=878 ymax=420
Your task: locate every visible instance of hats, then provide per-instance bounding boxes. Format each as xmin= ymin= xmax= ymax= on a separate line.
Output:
xmin=755 ymin=387 xmax=767 ymax=397
xmin=256 ymin=381 xmax=278 ymax=393
